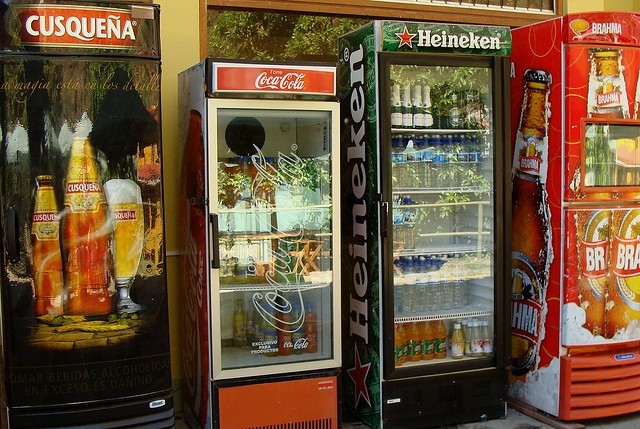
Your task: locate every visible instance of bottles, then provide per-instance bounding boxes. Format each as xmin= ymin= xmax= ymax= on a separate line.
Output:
xmin=446 ymin=134 xmax=456 ymax=188
xmin=512 ymin=68 xmax=556 ymax=377
xmin=449 ymin=89 xmax=484 ymax=129
xmin=468 ymin=133 xmax=482 ymax=185
xmin=393 ymin=195 xmax=403 ymax=253
xmin=408 ymin=322 xmax=423 ymax=361
xmin=413 ymin=84 xmax=424 ymax=128
xmin=428 ymin=259 xmax=442 ymax=312
xmin=461 ymin=320 xmax=467 ymax=339
xmin=418 ymin=138 xmax=431 ymax=187
xmin=398 ymin=324 xmax=407 ymax=362
xmin=391 ymin=140 xmax=397 ymax=187
xmin=304 ymin=303 xmax=318 ymax=353
xmin=404 ymin=257 xmax=415 ymax=314
xmin=464 ymin=322 xmax=473 ymax=357
xmin=218 ymin=155 xmax=316 ymax=208
xmin=61 ymin=137 xmax=118 ymax=317
xmin=421 ymin=322 xmax=435 ymax=360
xmin=232 ymin=299 xmax=247 ymax=348
xmin=102 ymin=178 xmax=148 ymax=316
xmin=451 ymin=251 xmax=466 ymax=309
xmin=402 ymin=82 xmax=413 ymax=129
xmin=458 ymin=133 xmax=470 ymax=187
xmin=575 ymin=48 xmax=640 ymax=336
xmin=277 ymin=237 xmax=290 ymax=285
xmin=403 ymin=195 xmax=416 ymax=252
xmin=451 ymin=323 xmax=464 ymax=359
xmin=395 ymin=325 xmax=404 ymax=366
xmin=278 ymin=310 xmax=291 ymax=356
xmin=235 ymin=258 xmax=250 ymax=284
xmin=465 ymin=324 xmax=472 ymax=357
xmin=481 ymin=321 xmax=492 ymax=356
xmin=441 ymin=254 xmax=453 ymax=310
xmin=258 ymin=305 xmax=269 ymax=353
xmin=602 ymin=101 xmax=640 ymax=339
xmin=291 ymin=237 xmax=304 ymax=284
xmin=246 ymin=299 xmax=257 ymax=346
xmin=391 ymin=81 xmax=402 ymax=128
xmin=29 ymin=174 xmax=64 ymax=320
xmin=395 ymin=137 xmax=406 ymax=187
xmin=470 ymin=322 xmax=484 ymax=357
xmin=291 ymin=303 xmax=304 ymax=355
xmin=424 ymin=85 xmax=434 ymax=129
xmin=395 ymin=262 xmax=403 ymax=314
xmin=263 ymin=304 xmax=278 ymax=357
xmin=434 ymin=319 xmax=447 ymax=359
xmin=465 ymin=281 xmax=472 ymax=305
xmin=430 ymin=135 xmax=445 ymax=187
xmin=406 ymin=136 xmax=419 ymax=188
xmin=448 ymin=319 xmax=457 ymax=350
xmin=416 ymin=257 xmax=427 ymax=313
xmin=434 ymin=102 xmax=446 ymax=127
